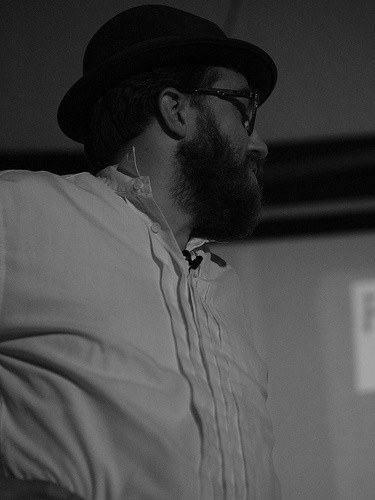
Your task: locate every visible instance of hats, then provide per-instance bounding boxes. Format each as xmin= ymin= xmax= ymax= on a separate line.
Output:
xmin=57 ymin=2 xmax=280 ymax=144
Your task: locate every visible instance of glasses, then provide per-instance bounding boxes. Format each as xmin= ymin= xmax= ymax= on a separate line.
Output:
xmin=171 ymin=84 xmax=260 ymax=137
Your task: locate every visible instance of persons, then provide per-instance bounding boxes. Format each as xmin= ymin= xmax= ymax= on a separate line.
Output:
xmin=0 ymin=4 xmax=283 ymax=499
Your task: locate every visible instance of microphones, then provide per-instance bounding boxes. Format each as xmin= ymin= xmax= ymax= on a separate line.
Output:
xmin=183 ymin=250 xmax=204 ymax=270
